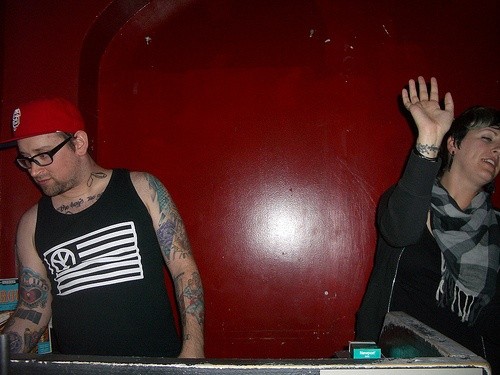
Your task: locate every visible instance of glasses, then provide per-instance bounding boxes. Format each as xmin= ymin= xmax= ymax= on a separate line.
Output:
xmin=15 ymin=133 xmax=72 ymax=171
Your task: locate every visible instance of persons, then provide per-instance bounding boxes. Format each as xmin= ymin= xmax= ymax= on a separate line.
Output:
xmin=354 ymin=75 xmax=500 ymax=362
xmin=0 ymin=98 xmax=205 ymax=359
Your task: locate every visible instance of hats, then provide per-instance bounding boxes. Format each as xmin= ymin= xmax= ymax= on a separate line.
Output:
xmin=1 ymin=92 xmax=86 ymax=145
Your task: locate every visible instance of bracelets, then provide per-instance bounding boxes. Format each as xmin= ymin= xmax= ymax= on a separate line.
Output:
xmin=414 ymin=148 xmax=439 ymax=161
